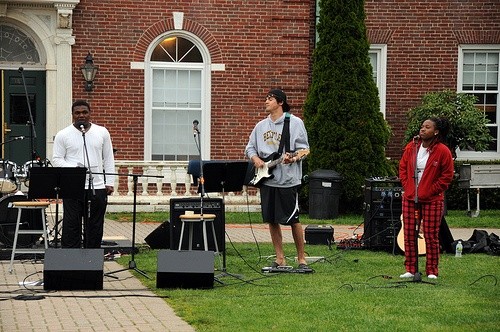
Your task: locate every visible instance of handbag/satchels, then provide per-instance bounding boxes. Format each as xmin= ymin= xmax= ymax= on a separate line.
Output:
xmin=446 ymin=239 xmax=479 ymax=254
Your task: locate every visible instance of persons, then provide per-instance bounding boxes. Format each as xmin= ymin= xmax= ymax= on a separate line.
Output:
xmin=245 ymin=90 xmax=310 ymax=271
xmin=52 ymin=100 xmax=117 ymax=248
xmin=400 ymin=117 xmax=454 ymax=279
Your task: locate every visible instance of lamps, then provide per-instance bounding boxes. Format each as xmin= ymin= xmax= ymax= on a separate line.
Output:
xmin=79 ymin=51 xmax=97 ymax=91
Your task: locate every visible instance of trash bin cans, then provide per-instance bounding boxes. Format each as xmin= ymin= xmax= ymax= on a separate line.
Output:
xmin=305 ymin=170 xmax=343 ymax=220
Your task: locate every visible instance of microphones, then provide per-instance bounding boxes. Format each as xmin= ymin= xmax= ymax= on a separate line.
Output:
xmin=192 ymin=120 xmax=200 ymax=136
xmin=79 ymin=120 xmax=87 ymax=134
xmin=413 ymin=135 xmax=421 ymax=140
xmin=5 ymin=135 xmax=25 ymax=143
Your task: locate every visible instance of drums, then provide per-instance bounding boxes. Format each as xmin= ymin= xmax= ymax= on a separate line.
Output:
xmin=0 ymin=161 xmax=19 ymax=194
xmin=23 ymin=161 xmax=50 ymax=188
xmin=0 ymin=191 xmax=47 ymax=249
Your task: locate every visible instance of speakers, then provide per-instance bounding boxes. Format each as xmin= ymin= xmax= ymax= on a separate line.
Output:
xmin=43 ymin=247 xmax=105 ymax=290
xmin=170 ymin=198 xmax=225 ymax=252
xmin=145 ymin=220 xmax=172 ymax=250
xmin=154 ymin=251 xmax=215 ymax=289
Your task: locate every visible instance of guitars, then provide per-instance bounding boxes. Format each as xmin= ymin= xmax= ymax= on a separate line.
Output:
xmin=246 ymin=147 xmax=310 ymax=188
xmin=397 ymin=213 xmax=427 ymax=255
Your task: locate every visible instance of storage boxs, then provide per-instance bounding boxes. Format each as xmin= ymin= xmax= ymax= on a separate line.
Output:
xmin=305 ymin=225 xmax=334 ymax=245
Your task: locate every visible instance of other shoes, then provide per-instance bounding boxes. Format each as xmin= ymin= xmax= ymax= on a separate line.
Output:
xmin=270 ymin=261 xmax=287 ymax=268
xmin=428 ymin=274 xmax=437 ymax=279
xmin=299 ymin=263 xmax=308 ymax=269
xmin=400 ymin=271 xmax=414 ymax=278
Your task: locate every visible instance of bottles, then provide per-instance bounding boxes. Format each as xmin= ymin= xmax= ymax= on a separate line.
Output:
xmin=456 ymin=241 xmax=463 ymax=257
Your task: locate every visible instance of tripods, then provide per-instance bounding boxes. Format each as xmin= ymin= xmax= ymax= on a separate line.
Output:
xmin=397 ymin=168 xmax=437 ymax=285
xmin=105 ymin=177 xmax=153 ymax=281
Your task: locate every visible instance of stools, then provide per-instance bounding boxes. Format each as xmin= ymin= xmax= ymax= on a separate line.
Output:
xmin=9 ymin=202 xmax=50 ymax=273
xmin=178 ymin=214 xmax=222 ymax=270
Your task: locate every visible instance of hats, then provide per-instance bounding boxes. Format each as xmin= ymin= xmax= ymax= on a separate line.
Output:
xmin=268 ymin=89 xmax=290 ymax=112
